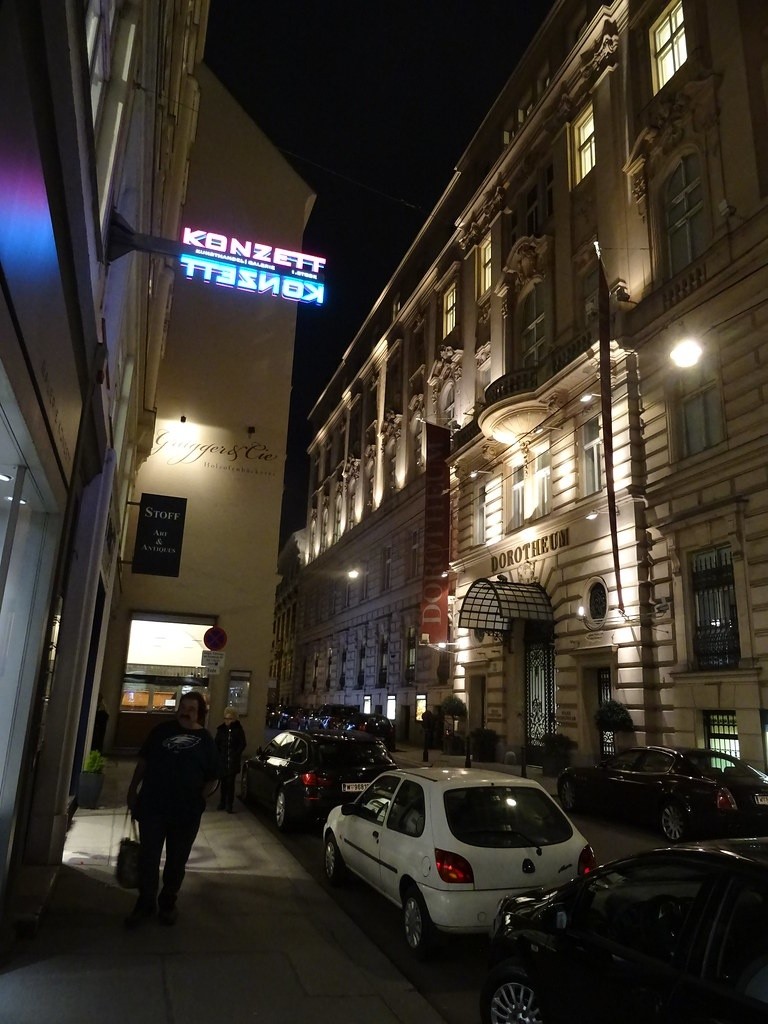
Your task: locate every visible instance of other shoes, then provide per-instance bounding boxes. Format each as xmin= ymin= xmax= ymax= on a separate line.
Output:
xmin=226 ymin=803 xmax=232 ymax=813
xmin=157 ymin=905 xmax=179 ymax=927
xmin=217 ymin=801 xmax=226 ymax=811
xmin=122 ymin=903 xmax=156 ymax=928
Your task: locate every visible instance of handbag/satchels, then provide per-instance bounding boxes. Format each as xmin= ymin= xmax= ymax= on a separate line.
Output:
xmin=117 ymin=808 xmax=139 ymax=888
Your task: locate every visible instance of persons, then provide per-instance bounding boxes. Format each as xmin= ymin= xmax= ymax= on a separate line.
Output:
xmin=91 ymin=693 xmax=109 ymax=755
xmin=125 ymin=691 xmax=217 ymax=930
xmin=215 ymin=707 xmax=248 ymax=812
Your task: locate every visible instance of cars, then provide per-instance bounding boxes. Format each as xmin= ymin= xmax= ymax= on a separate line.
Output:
xmin=241 ymin=731 xmax=400 ymax=831
xmin=479 ymin=836 xmax=768 ymax=1024
xmin=556 ymin=745 xmax=768 ymax=844
xmin=323 ymin=766 xmax=599 ymax=956
xmin=266 ymin=703 xmax=396 ymax=752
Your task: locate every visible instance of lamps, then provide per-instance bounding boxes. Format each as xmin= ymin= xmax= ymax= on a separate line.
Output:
xmin=585 ymin=509 xmax=620 ymax=521
xmin=180 ymin=416 xmax=187 ymax=424
xmin=410 ymin=542 xmax=425 ymax=549
xmin=418 ymin=633 xmax=458 ymax=655
xmin=534 ymin=426 xmax=563 ymax=434
xmin=617 ymin=288 xmax=639 ymax=306
xmin=575 ymin=606 xmax=591 ymax=624
xmin=613 ymin=603 xmax=669 ymax=624
xmin=247 ymin=426 xmax=256 ymax=439
xmin=440 ymin=569 xmax=465 ymax=578
xmin=440 ymin=489 xmax=451 ymax=496
xmin=385 ymin=556 xmax=401 ymax=564
xmin=468 ymin=470 xmax=494 ymax=478
xmin=579 ymin=393 xmax=614 ymax=402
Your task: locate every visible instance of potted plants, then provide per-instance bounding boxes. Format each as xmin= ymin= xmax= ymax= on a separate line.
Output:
xmin=539 ymin=733 xmax=568 ymax=778
xmin=438 ymin=696 xmax=467 ymax=754
xmin=467 ymin=727 xmax=498 ymax=762
xmin=77 ymin=749 xmax=106 ymax=810
